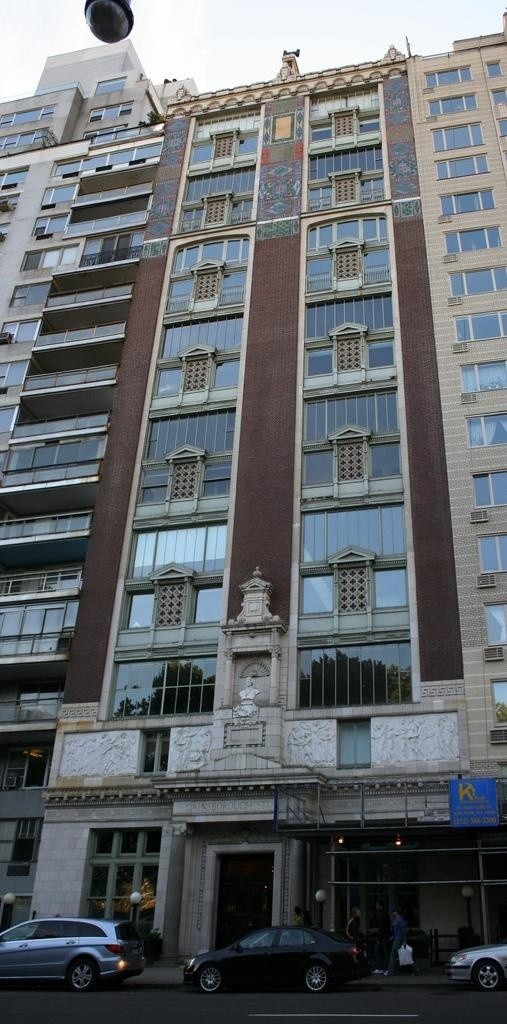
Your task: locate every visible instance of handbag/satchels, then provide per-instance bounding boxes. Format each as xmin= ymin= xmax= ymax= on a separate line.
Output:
xmin=398 ymin=947 xmax=414 ymax=966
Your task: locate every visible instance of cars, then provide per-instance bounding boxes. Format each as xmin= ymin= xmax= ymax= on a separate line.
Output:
xmin=1 ymin=916 xmax=145 ymax=991
xmin=182 ymin=926 xmax=362 ymax=992
xmin=445 ymin=940 xmax=506 ymax=991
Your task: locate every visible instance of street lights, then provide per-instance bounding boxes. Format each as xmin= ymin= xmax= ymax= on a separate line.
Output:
xmin=84 ymin=0 xmax=134 ymax=43
xmin=461 ymin=885 xmax=474 ymax=926
xmin=128 ymin=892 xmax=142 ymax=923
xmin=315 ymin=889 xmax=326 ymax=928
xmin=3 ymin=892 xmax=15 ymax=928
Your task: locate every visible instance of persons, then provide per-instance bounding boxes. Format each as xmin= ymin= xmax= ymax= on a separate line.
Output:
xmin=303 ymin=911 xmax=313 ymax=925
xmin=346 ymin=906 xmax=363 ymax=943
xmin=382 ymin=903 xmax=421 ymax=977
xmin=239 ymin=676 xmax=260 ymax=701
xmin=292 ymin=906 xmax=304 ymax=925
xmin=370 ymin=899 xmax=391 ymax=975
